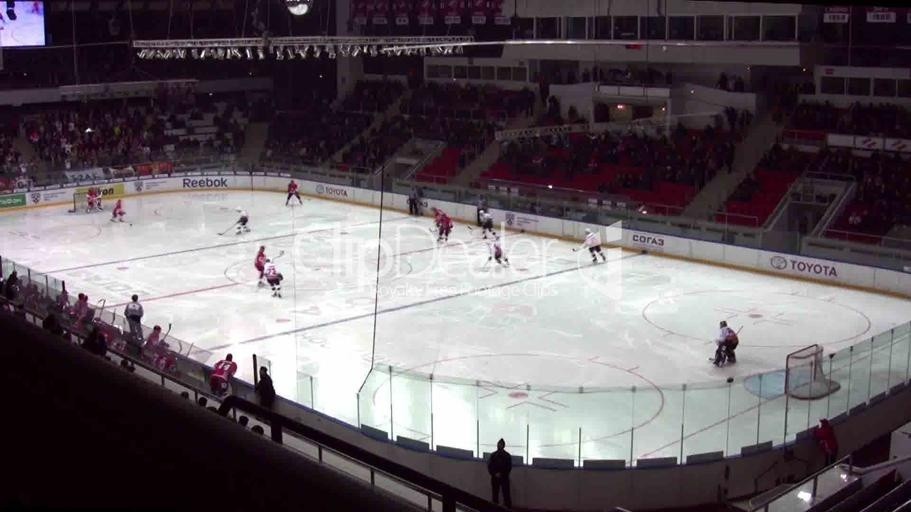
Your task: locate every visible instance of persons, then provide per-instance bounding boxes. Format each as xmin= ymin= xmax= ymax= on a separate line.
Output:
xmin=263 ymin=68 xmax=911 ymax=239
xmin=234 ymin=207 xmax=252 ymax=234
xmin=484 ymin=239 xmax=512 ymax=267
xmin=711 ymin=321 xmax=739 ymax=367
xmin=2 ymin=84 xmax=249 ymax=193
xmin=85 ymin=185 xmax=126 ymax=223
xmin=815 ymin=419 xmax=838 ymax=466
xmin=488 ymin=438 xmax=512 ymax=509
xmin=254 ymin=246 xmax=282 ymax=299
xmin=0 ymin=267 xmax=278 ymax=435
xmin=581 ymin=226 xmax=607 ymax=263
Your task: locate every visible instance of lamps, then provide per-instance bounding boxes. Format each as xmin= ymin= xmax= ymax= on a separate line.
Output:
xmin=285 ymin=0 xmax=314 ymax=18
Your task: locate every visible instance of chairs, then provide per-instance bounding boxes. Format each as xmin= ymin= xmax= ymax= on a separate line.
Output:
xmin=1 ymin=74 xmax=911 ymax=244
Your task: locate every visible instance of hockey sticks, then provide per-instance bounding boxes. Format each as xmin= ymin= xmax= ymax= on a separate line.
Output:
xmin=572 ymin=246 xmax=584 ymax=252
xmin=709 ymin=328 xmax=743 ymax=361
xmin=270 ymin=250 xmax=285 ymax=264
xmin=218 ymin=224 xmax=238 ymax=235
